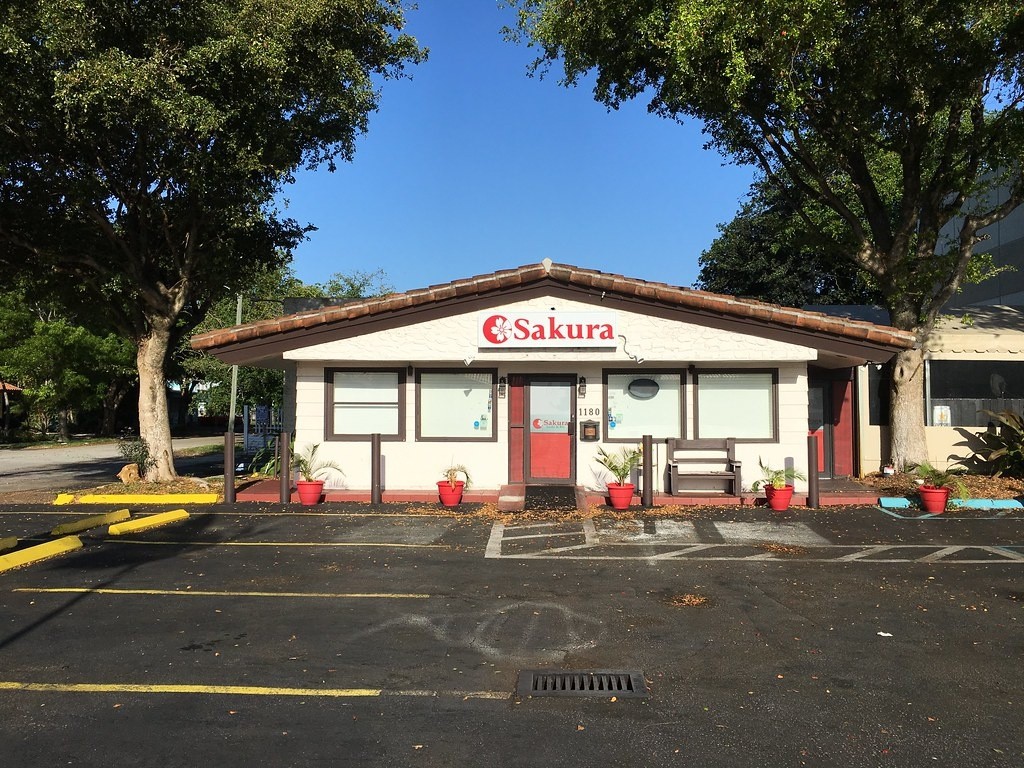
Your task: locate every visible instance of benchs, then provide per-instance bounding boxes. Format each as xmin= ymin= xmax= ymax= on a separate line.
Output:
xmin=667 ymin=437 xmax=742 ymax=497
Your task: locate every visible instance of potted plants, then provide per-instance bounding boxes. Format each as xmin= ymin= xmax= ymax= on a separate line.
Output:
xmin=593 ymin=441 xmax=644 ymax=508
xmin=752 ymin=454 xmax=807 ymax=510
xmin=904 ymin=457 xmax=961 ymax=512
xmin=437 ymin=464 xmax=474 ymax=506
xmin=289 ymin=444 xmax=346 ymax=505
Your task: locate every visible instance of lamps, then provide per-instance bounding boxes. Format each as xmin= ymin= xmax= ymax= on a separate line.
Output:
xmin=463 ymin=341 xmax=479 ymax=366
xmin=619 ymin=334 xmax=645 ymax=364
